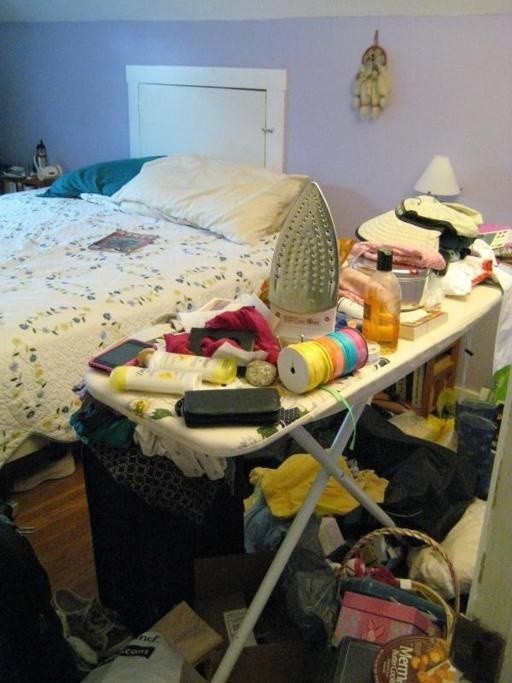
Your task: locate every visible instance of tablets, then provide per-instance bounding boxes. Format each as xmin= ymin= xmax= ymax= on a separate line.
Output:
xmin=89 ymin=337 xmax=158 ymax=374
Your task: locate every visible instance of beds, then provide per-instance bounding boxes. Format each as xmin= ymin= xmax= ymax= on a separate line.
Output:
xmin=0 ymin=186 xmax=281 ymax=497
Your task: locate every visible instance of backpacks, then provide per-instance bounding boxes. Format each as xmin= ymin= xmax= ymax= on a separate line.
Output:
xmin=0 ymin=504 xmax=80 ymax=682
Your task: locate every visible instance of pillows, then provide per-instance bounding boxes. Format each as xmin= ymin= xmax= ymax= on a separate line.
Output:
xmin=38 ymin=156 xmax=309 ymax=244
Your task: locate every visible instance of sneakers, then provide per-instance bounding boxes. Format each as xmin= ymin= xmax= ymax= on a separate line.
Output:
xmin=51 ymin=586 xmax=134 ymax=661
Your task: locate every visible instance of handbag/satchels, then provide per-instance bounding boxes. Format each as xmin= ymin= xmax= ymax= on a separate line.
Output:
xmin=354 ymin=193 xmax=485 ymax=264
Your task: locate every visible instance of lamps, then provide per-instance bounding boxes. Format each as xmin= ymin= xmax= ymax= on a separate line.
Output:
xmin=412 ymin=154 xmax=461 ymax=201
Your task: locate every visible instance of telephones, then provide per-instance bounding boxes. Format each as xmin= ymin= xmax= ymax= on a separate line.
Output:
xmin=31 ymin=140 xmax=62 ymax=182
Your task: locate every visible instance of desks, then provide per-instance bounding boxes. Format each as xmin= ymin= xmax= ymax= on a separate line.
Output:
xmin=82 ymin=279 xmax=512 ymax=682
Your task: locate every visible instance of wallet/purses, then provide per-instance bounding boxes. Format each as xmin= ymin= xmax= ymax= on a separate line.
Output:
xmin=176 ymin=388 xmax=281 ymax=428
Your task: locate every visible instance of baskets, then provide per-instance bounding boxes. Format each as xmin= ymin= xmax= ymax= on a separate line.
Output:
xmin=336 ymin=527 xmax=462 ymax=644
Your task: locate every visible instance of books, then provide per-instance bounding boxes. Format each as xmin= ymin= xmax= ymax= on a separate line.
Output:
xmin=88 ymin=230 xmax=160 ymax=255
xmin=395 ymin=307 xmax=448 ymax=340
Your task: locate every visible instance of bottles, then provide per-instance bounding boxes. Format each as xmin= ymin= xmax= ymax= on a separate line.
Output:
xmin=361 ymin=247 xmax=404 ymax=355
